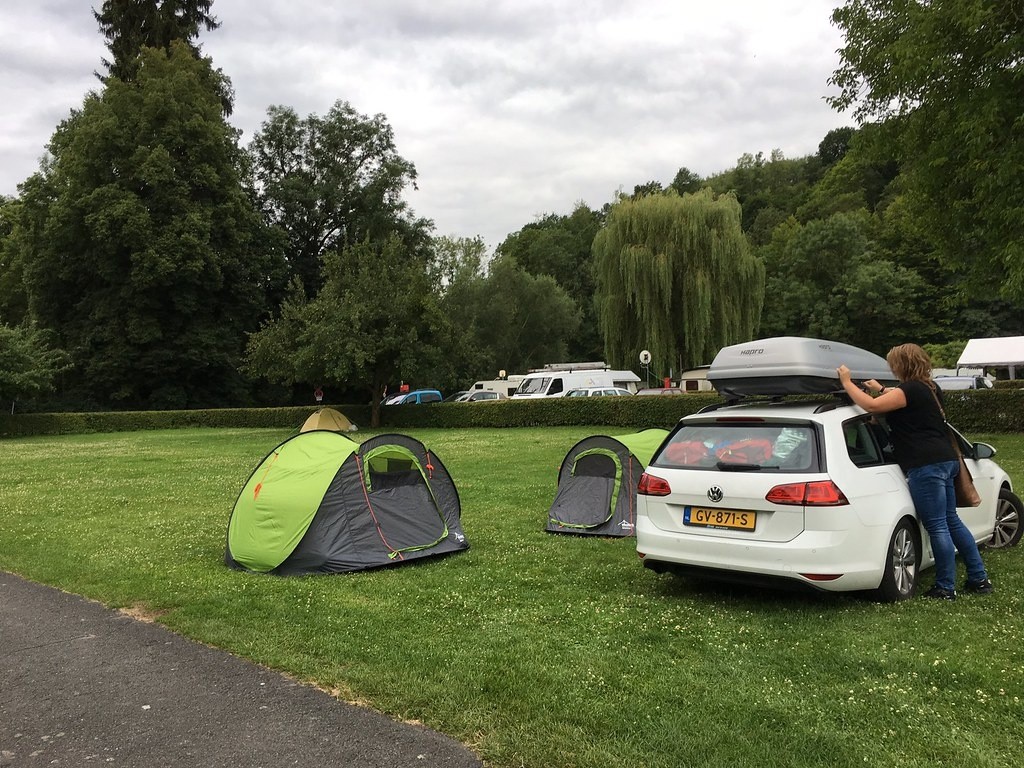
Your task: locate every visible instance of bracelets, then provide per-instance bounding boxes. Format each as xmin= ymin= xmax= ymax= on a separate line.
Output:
xmin=879 ymin=387 xmax=884 ymax=394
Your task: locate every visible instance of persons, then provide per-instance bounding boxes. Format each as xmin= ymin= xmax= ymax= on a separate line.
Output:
xmin=837 ymin=344 xmax=994 ymax=601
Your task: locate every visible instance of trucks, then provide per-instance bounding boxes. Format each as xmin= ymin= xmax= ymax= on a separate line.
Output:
xmin=468 ymin=375 xmax=526 ymax=398
xmin=509 ymin=362 xmax=641 ymax=400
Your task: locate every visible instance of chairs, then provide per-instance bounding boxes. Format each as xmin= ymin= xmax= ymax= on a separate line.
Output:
xmin=856 ymin=424 xmax=896 ymax=463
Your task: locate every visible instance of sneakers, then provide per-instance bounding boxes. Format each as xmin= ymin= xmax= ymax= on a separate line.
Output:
xmin=921 ymin=585 xmax=956 ymax=602
xmin=959 ymin=579 xmax=992 ymax=594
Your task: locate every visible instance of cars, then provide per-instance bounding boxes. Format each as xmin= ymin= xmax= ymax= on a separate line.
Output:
xmin=634 ymin=388 xmax=688 ymax=395
xmin=465 ymin=391 xmax=507 ymax=402
xmin=635 ymin=335 xmax=1024 ymax=603
xmin=443 ymin=391 xmax=469 ymax=403
xmin=385 ymin=394 xmax=406 ymax=405
xmin=930 ymin=375 xmax=995 ymax=391
xmin=564 ymin=387 xmax=635 ymax=397
xmin=455 ymin=394 xmax=472 ymax=403
xmin=399 ymin=390 xmax=442 ymax=404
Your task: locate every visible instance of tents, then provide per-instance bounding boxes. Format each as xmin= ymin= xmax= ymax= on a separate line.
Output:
xmin=223 ymin=429 xmax=470 ymax=573
xmin=544 ymin=428 xmax=673 ymax=536
xmin=955 ymin=335 xmax=1024 ymax=381
xmin=300 ymin=408 xmax=355 ymax=433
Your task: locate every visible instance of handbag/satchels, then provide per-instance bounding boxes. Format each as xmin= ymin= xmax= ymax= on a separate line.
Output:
xmin=950 ymin=433 xmax=981 ymax=507
